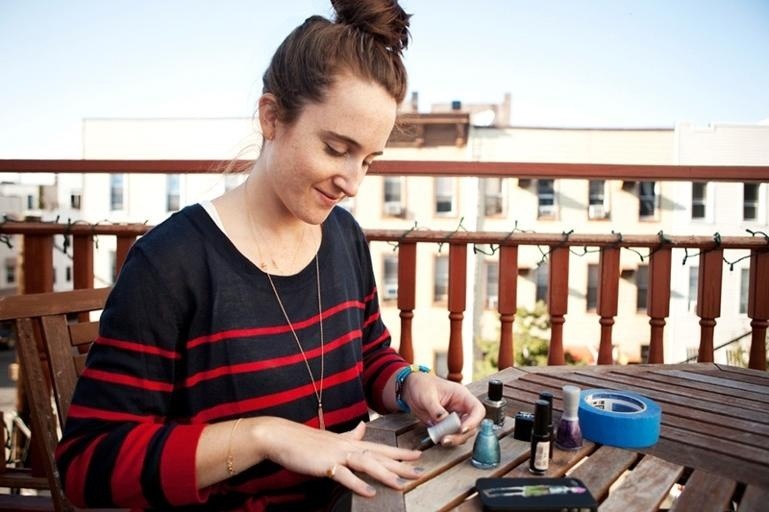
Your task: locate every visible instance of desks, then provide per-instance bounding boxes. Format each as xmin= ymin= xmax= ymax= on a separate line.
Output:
xmin=351 ymin=361 xmax=767 ymax=511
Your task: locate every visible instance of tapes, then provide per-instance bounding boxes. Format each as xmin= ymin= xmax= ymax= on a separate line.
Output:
xmin=578 ymin=389 xmax=662 ymax=448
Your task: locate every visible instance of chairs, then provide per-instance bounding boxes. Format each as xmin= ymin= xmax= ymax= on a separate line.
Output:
xmin=0 ymin=286 xmax=120 ymax=511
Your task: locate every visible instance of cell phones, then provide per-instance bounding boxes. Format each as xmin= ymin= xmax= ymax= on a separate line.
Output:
xmin=475 ymin=476 xmax=598 ymax=512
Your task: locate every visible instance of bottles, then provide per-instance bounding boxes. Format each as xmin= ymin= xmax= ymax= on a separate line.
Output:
xmin=540 ymin=392 xmax=555 ymax=458
xmin=481 ymin=379 xmax=508 ymax=427
xmin=526 ymin=398 xmax=550 ymax=475
xmin=470 ymin=419 xmax=501 ymax=471
xmin=554 ymin=384 xmax=583 ymax=452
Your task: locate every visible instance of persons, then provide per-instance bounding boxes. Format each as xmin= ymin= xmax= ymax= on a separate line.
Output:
xmin=54 ymin=0 xmax=490 ymax=512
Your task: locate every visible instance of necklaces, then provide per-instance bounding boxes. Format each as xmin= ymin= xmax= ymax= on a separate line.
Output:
xmin=224 ymin=418 xmax=242 ymax=481
xmin=244 ymin=180 xmax=325 ymax=431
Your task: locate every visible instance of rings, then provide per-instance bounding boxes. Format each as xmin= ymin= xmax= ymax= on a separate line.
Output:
xmin=345 ymin=452 xmax=354 ymax=467
xmin=326 ymin=461 xmax=340 ymax=481
xmin=363 ymin=448 xmax=370 ymax=457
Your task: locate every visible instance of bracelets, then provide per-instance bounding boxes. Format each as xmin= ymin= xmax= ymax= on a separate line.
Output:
xmin=393 ymin=364 xmax=436 ymax=413
